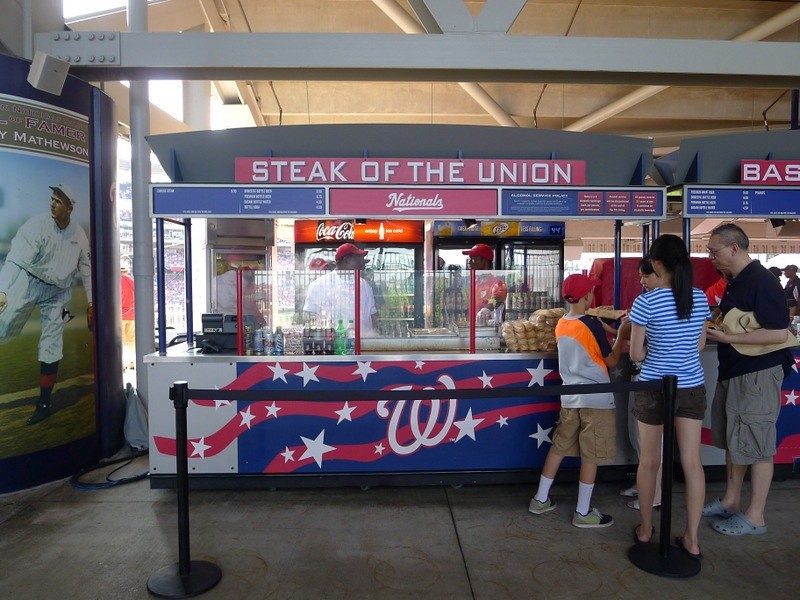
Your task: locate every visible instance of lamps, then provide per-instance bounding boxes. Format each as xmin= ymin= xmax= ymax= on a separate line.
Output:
xmin=27 ymin=50 xmax=70 ymax=96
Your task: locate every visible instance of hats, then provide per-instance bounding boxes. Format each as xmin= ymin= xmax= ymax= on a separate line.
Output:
xmin=462 ymin=244 xmax=494 ymax=260
xmin=769 ymin=267 xmax=782 ymax=276
xmin=364 ymin=259 xmax=370 ymax=263
xmin=49 ymin=182 xmax=76 ymax=205
xmin=562 ymin=274 xmax=602 ymax=303
xmin=309 ymin=258 xmax=328 ymax=270
xmin=329 ymin=261 xmax=336 ymax=266
xmin=722 ymin=307 xmax=799 ymax=356
xmin=334 ymin=243 xmax=368 ymax=261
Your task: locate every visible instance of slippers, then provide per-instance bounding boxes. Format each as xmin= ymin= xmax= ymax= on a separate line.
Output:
xmin=702 ymin=496 xmax=743 ymax=519
xmin=711 ymin=512 xmax=767 ymax=536
xmin=676 ymin=536 xmax=703 ymax=559
xmin=633 ymin=523 xmax=655 ymax=544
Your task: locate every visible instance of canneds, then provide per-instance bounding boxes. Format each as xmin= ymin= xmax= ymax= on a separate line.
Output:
xmin=253 ymin=330 xmax=273 ymax=355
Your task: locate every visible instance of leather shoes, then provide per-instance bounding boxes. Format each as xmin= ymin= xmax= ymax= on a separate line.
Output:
xmin=25 ymin=401 xmax=51 ymax=424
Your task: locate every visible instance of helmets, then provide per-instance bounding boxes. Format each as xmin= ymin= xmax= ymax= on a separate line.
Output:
xmin=781 ymin=264 xmax=798 ymax=272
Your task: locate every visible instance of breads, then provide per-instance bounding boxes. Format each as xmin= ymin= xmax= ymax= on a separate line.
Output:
xmin=502 ymin=307 xmax=564 ymax=351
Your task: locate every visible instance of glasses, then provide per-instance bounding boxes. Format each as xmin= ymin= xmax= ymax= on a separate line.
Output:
xmin=705 ymin=241 xmax=740 ymax=257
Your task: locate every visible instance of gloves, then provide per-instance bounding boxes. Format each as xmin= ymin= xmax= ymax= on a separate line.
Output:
xmin=476 ymin=308 xmax=491 ymax=326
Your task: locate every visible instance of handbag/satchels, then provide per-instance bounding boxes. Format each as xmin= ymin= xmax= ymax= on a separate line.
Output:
xmin=627 ymin=346 xmax=648 ymax=375
xmin=124 ymin=383 xmax=149 ymax=451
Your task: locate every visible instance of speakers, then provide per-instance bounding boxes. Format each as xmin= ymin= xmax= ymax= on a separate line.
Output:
xmin=27 ymin=50 xmax=77 ymax=97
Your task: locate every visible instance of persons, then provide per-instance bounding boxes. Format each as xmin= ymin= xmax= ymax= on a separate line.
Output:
xmin=121 ymin=263 xmax=135 ymax=349
xmin=302 ymin=243 xmax=377 ymax=339
xmin=0 ymin=182 xmax=93 ymax=426
xmin=769 ymin=265 xmax=800 ymax=320
xmin=628 ymin=234 xmax=712 ymax=561
xmin=597 ymin=255 xmax=663 ymax=510
xmin=529 ymin=274 xmax=631 ymax=528
xmin=463 ymin=244 xmax=508 ymax=328
xmin=706 ymin=222 xmax=790 ymax=537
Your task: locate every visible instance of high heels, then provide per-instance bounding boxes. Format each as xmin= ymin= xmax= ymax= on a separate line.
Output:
xmin=618 ymin=488 xmax=638 ymax=498
xmin=626 ymin=499 xmax=661 ymax=512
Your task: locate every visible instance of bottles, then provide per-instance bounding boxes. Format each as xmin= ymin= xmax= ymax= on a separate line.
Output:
xmin=335 ymin=319 xmax=355 ymax=355
xmin=504 ymin=312 xmax=532 ymax=321
xmin=304 ymin=320 xmax=335 ymax=355
xmin=436 ymin=312 xmax=466 ymax=325
xmin=374 ymin=322 xmax=411 ymax=337
xmin=244 ymin=325 xmax=254 ymax=356
xmin=439 ymin=294 xmax=466 ymax=310
xmin=505 ymin=291 xmax=554 ymax=309
xmin=274 ymin=326 xmax=284 ymax=356
xmin=375 ymin=303 xmax=414 ymax=318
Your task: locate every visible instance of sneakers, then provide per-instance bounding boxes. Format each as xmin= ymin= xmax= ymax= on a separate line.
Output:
xmin=528 ymin=496 xmax=557 ymax=514
xmin=572 ymin=505 xmax=614 ymax=528
